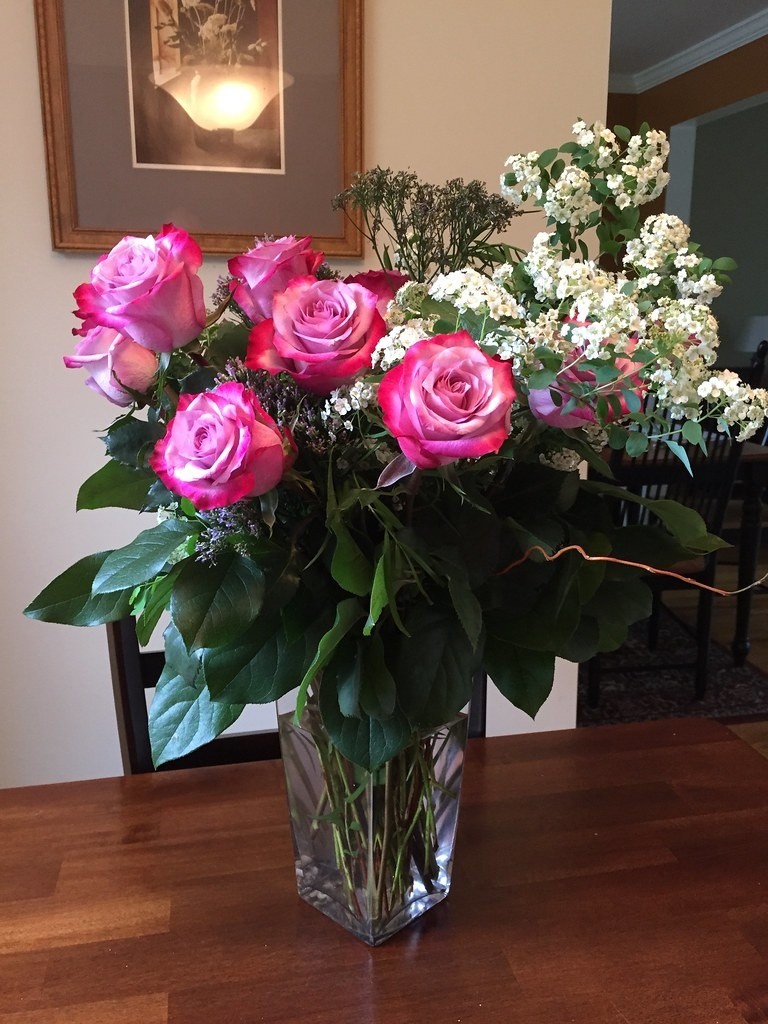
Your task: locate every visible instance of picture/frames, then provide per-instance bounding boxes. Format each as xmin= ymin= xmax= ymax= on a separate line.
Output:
xmin=34 ymin=0 xmax=368 ymax=259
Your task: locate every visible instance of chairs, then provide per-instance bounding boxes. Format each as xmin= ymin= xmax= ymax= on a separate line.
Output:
xmin=710 ymin=340 xmax=768 ymax=444
xmin=606 ymin=385 xmax=747 ymax=673
xmin=104 ymin=613 xmax=285 ymax=774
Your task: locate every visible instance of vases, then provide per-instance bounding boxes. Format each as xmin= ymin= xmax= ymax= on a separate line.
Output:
xmin=278 ymin=669 xmax=467 ymax=947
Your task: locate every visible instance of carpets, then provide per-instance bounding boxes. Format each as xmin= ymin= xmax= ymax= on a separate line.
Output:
xmin=578 ymin=595 xmax=766 ymax=728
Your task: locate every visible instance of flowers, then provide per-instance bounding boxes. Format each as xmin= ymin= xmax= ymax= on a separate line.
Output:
xmin=152 ymin=0 xmax=269 ymax=65
xmin=21 ymin=119 xmax=767 ymax=931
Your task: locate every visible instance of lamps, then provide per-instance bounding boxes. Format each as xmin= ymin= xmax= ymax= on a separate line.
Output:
xmin=151 ymin=63 xmax=294 ymax=152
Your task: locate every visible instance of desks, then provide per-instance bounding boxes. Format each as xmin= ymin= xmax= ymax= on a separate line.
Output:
xmin=615 ymin=426 xmax=768 ymax=671
xmin=2 ymin=713 xmax=766 ymax=1023
